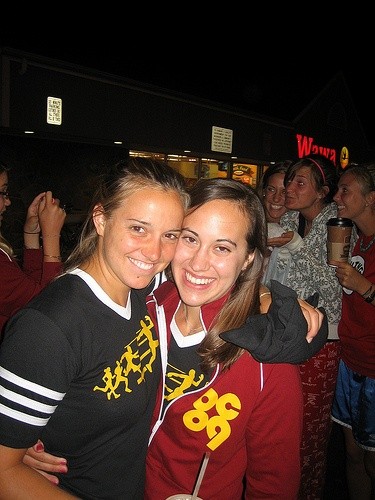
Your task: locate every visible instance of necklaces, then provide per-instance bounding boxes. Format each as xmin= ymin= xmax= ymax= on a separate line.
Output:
xmin=360 ymin=235 xmax=375 ymax=252
xmin=185 ymin=316 xmax=202 ymax=337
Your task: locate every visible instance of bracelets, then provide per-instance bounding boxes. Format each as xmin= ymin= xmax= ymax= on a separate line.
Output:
xmin=361 ymin=283 xmax=375 ymax=303
xmin=24 ymin=231 xmax=40 ymax=234
xmin=259 ymin=292 xmax=271 ymax=298
xmin=43 ymin=255 xmax=64 ymax=261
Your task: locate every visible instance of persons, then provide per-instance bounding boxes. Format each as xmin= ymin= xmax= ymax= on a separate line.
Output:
xmin=250 ymin=154 xmax=374 ymax=500
xmin=23 ymin=178 xmax=303 ymax=500
xmin=0 ymin=164 xmax=93 ymax=339
xmin=0 ymin=158 xmax=325 ymax=500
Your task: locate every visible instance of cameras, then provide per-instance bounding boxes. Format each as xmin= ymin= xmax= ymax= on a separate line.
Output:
xmin=40 ymin=196 xmax=55 ymax=205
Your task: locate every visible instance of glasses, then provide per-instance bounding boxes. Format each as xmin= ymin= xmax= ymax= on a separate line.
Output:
xmin=0 ymin=190 xmax=10 ymax=196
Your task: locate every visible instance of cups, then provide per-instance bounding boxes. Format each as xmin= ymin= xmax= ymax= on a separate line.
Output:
xmin=326 ymin=218 xmax=354 ymax=268
xmin=166 ymin=494 xmax=203 ymax=500
